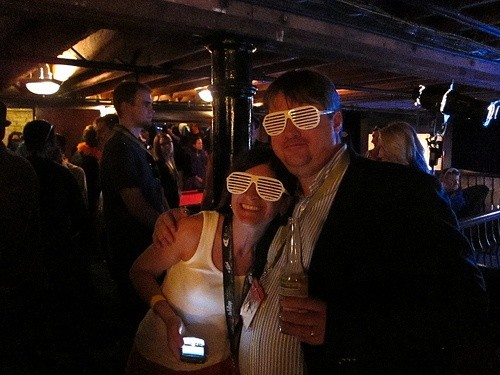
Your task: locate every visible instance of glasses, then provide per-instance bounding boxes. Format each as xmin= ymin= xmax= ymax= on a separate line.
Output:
xmin=262 ymin=105 xmax=335 ymax=136
xmin=226 ymin=172 xmax=289 ymax=201
xmin=45 ymin=121 xmax=56 ymax=143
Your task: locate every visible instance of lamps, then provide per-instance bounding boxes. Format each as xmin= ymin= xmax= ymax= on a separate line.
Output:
xmin=24 ymin=64 xmax=64 ymax=98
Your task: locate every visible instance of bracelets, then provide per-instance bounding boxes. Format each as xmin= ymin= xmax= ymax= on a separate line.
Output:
xmin=149 ymin=295 xmax=166 ymax=313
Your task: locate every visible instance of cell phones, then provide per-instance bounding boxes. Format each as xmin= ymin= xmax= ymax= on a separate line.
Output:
xmin=179 ymin=335 xmax=207 ymax=365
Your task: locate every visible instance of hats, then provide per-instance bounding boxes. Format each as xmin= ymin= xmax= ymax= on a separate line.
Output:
xmin=0 ymin=102 xmax=11 ymax=127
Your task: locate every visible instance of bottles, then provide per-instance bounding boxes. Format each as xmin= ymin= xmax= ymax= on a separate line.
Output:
xmin=279 ymin=217 xmax=309 ymax=333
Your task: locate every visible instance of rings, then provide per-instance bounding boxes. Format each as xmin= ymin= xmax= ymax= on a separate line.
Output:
xmin=309 ymin=327 xmax=315 ymax=337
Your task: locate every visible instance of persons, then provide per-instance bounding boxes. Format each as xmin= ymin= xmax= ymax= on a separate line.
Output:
xmin=0 ymin=72 xmax=500 ymax=374
